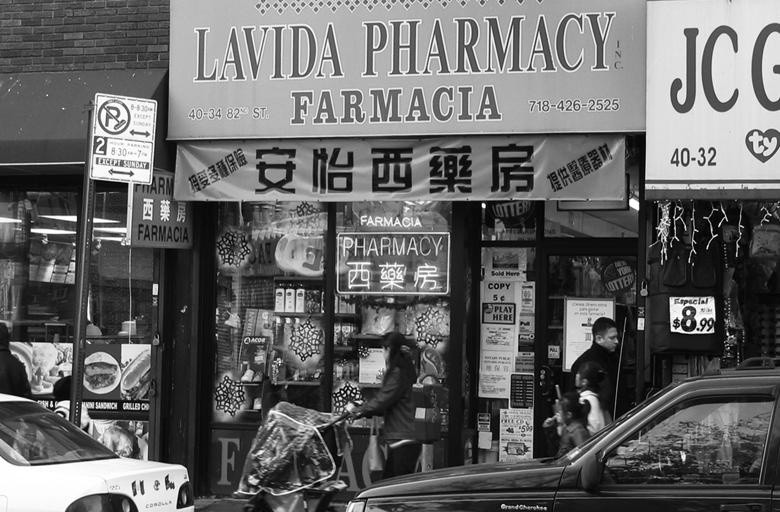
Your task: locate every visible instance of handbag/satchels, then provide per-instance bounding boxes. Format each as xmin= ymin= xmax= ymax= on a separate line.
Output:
xmin=368 ymin=415 xmax=385 ymax=472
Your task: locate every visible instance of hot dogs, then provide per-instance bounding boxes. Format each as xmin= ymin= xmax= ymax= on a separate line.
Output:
xmin=119 ymin=348 xmax=151 ymax=396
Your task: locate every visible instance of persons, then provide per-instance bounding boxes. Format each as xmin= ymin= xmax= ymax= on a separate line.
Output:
xmin=0 ymin=322 xmax=31 ymax=401
xmin=40 ymin=374 xmax=96 ymax=461
xmin=348 ymin=332 xmax=440 ymax=478
xmin=555 ymin=317 xmax=621 ymax=459
